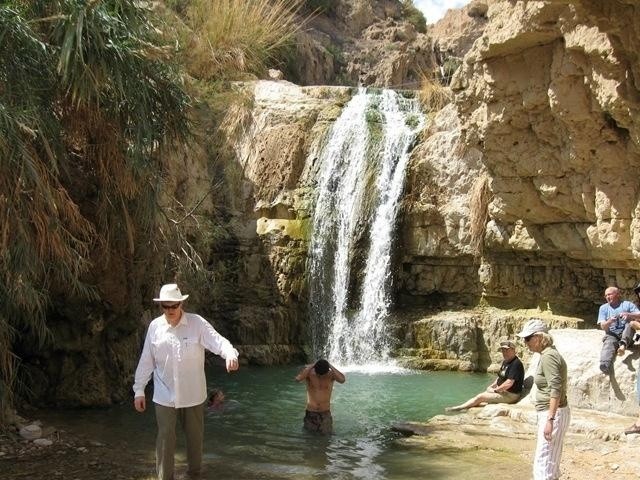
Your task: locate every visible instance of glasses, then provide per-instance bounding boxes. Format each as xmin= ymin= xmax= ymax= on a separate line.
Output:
xmin=525 ymin=335 xmax=534 ymax=342
xmin=161 ymin=302 xmax=180 ymax=309
xmin=604 ymin=293 xmax=615 ymax=298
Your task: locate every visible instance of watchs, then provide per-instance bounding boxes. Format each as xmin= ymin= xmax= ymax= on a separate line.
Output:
xmin=546 ymin=416 xmax=554 ymax=421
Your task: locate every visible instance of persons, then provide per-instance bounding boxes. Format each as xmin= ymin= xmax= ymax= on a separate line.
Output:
xmin=443 ymin=342 xmax=524 ymax=411
xmin=516 ymin=320 xmax=571 ymax=480
xmin=296 ymin=358 xmax=345 ymax=435
xmin=625 ymin=288 xmax=639 ymax=434
xmin=132 ymin=284 xmax=239 ymax=480
xmin=597 ymin=287 xmax=640 ymax=373
xmin=207 ymin=389 xmax=224 ymax=410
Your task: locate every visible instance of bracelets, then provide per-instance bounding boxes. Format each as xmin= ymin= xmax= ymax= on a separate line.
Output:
xmin=494 ymin=388 xmax=498 ymax=393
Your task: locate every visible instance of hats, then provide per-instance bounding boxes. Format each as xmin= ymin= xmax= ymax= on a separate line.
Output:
xmin=152 ymin=283 xmax=190 ymax=301
xmin=515 ymin=318 xmax=547 ymax=338
xmin=497 ymin=341 xmax=515 ymax=351
